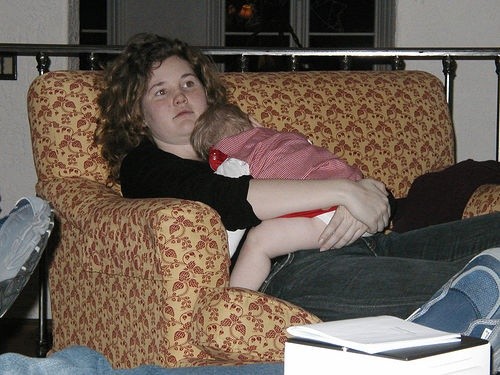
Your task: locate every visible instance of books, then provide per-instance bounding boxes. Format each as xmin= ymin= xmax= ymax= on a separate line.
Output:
xmin=284 ymin=333 xmax=491 ymax=375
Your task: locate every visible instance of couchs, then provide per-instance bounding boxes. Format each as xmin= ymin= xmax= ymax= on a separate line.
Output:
xmin=28 ymin=70 xmax=500 ymax=370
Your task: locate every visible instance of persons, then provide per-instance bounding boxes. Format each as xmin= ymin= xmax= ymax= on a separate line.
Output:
xmin=191 ymin=101 xmax=376 ymax=292
xmin=90 ymin=33 xmax=500 ymax=325
